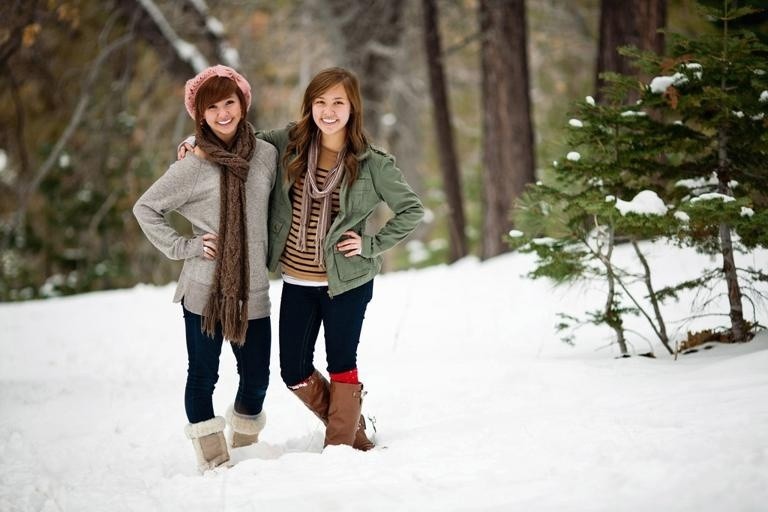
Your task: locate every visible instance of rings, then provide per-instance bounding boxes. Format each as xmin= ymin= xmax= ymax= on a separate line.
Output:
xmin=204 ymin=247 xmax=207 ymax=251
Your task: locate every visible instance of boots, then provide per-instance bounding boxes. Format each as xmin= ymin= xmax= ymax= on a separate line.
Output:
xmin=186 ymin=414 xmax=267 ymax=473
xmin=287 ymin=370 xmax=374 ymax=451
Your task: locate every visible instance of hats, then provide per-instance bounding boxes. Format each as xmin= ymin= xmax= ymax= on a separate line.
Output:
xmin=184 ymin=64 xmax=251 ymax=124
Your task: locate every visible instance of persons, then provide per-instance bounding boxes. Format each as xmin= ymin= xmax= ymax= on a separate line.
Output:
xmin=174 ymin=66 xmax=427 ymax=452
xmin=130 ymin=65 xmax=278 ymax=468
